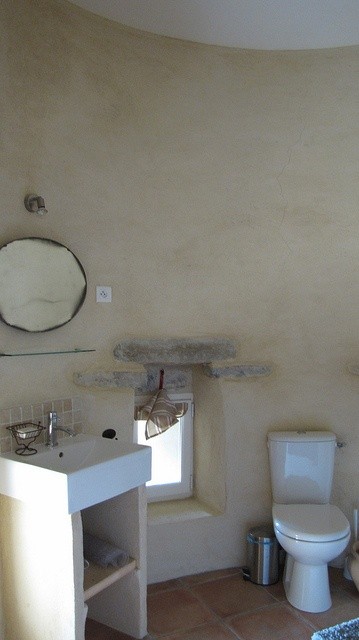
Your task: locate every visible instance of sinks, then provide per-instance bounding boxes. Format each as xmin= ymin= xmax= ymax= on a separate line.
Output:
xmin=0 ymin=431 xmax=152 ymax=515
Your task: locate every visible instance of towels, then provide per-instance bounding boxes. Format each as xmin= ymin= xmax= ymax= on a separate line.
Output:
xmin=136 ymin=389 xmax=186 ymax=440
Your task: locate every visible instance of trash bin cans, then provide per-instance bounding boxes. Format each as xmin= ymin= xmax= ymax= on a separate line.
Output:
xmin=240 ymin=525 xmax=281 ymax=585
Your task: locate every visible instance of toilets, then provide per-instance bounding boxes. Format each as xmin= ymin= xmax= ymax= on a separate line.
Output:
xmin=267 ymin=430 xmax=351 ymax=615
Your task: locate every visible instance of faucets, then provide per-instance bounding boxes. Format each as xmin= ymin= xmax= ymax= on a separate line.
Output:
xmin=44 ymin=412 xmax=77 ymax=447
